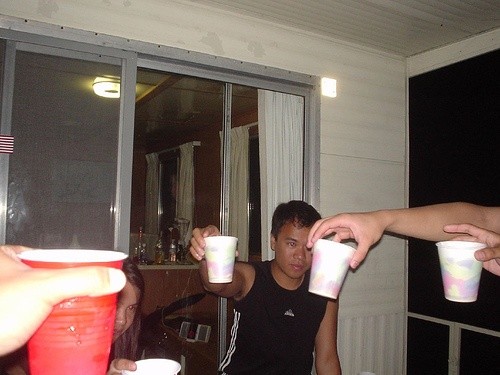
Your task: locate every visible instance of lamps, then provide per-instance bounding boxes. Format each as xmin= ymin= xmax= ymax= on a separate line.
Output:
xmin=92 ymin=76 xmax=121 ymax=98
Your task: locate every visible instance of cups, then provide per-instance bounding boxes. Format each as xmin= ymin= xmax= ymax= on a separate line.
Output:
xmin=205 ymin=236 xmax=238 ymax=284
xmin=436 ymin=240 xmax=487 ymax=303
xmin=307 ymin=238 xmax=357 ymax=300
xmin=122 ymin=358 xmax=182 ymax=375
xmin=19 ymin=248 xmax=130 ymax=375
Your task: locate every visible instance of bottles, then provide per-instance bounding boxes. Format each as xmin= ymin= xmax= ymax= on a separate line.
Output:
xmin=133 ymin=226 xmax=148 ymax=267
xmin=155 ymin=230 xmax=177 ymax=264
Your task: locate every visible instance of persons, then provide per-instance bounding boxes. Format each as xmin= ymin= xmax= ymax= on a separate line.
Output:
xmin=306 ymin=203 xmax=500 ymax=277
xmin=190 ymin=200 xmax=342 ymax=375
xmin=0 ymin=246 xmax=126 ymax=356
xmin=10 ymin=260 xmax=144 ymax=375
